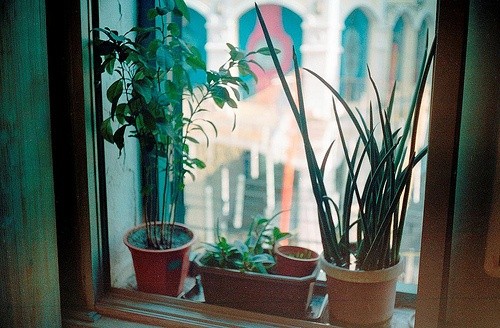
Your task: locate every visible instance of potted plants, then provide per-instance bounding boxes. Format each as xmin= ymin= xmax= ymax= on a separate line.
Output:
xmin=194 ymin=208 xmax=320 ymax=317
xmin=91 ymin=0 xmax=280 ymax=298
xmin=252 ymin=1 xmax=436 ymax=328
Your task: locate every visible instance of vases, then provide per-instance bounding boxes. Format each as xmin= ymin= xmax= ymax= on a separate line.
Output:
xmin=275 ymin=245 xmax=318 ymax=278
